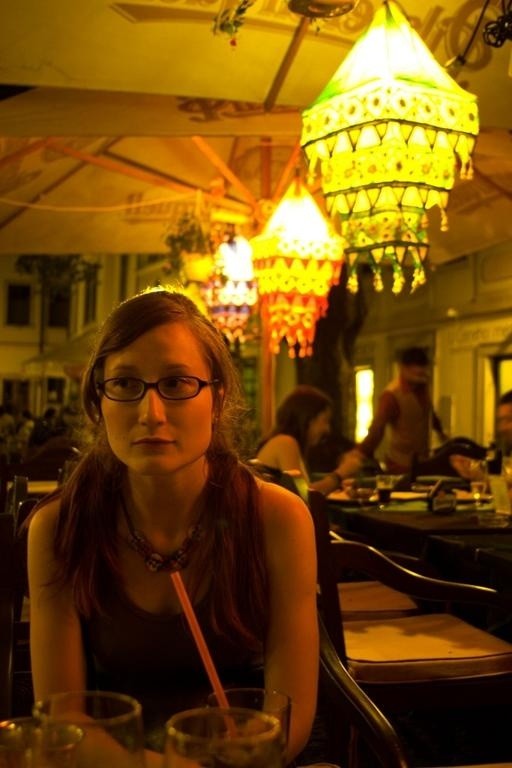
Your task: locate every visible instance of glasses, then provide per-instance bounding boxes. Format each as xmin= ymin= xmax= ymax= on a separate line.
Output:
xmin=96 ymin=374 xmax=224 ymax=403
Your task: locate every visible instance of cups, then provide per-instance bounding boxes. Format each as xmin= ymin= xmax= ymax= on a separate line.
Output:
xmin=378 ymin=472 xmax=399 ymax=506
xmin=470 ymin=460 xmax=487 ymax=510
xmin=0 ymin=692 xmax=147 ymax=768
xmin=165 ymin=685 xmax=290 ymax=767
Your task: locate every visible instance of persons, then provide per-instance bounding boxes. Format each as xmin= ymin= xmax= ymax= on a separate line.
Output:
xmin=2 ymin=404 xmax=32 ymax=460
xmin=254 ymin=385 xmax=365 ymax=503
xmin=355 ymin=346 xmax=450 ymax=475
xmin=29 ymin=407 xmax=57 ymax=455
xmin=28 ymin=291 xmax=318 ymax=768
xmin=449 ymin=388 xmax=512 ymax=488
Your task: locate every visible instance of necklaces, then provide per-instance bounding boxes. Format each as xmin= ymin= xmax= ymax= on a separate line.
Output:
xmin=118 ymin=503 xmax=215 ymax=574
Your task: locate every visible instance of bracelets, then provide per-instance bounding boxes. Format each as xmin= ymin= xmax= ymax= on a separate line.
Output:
xmin=327 ymin=471 xmax=341 ymax=486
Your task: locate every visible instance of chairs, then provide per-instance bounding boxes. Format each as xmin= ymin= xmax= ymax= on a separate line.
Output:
xmin=4 ymin=473 xmax=38 ymax=715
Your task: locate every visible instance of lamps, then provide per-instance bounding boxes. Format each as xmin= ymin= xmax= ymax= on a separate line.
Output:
xmin=248 ymin=179 xmax=346 ymax=359
xmin=298 ymin=2 xmax=480 ymax=298
